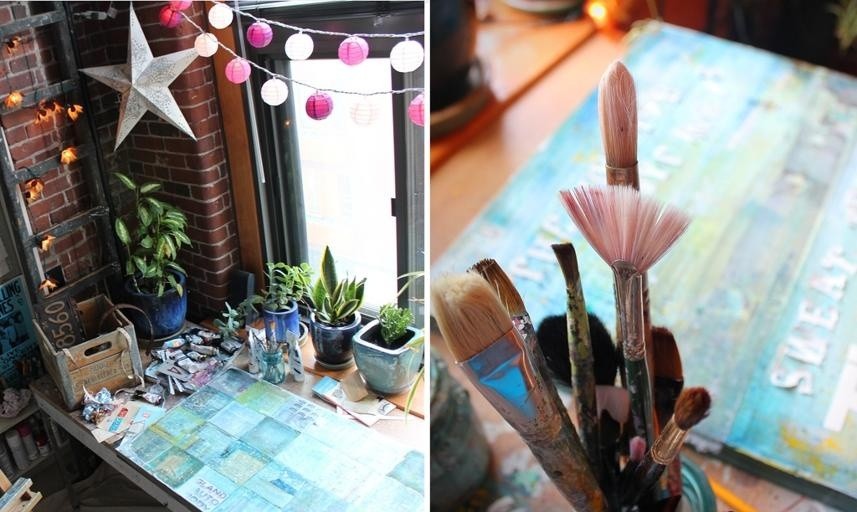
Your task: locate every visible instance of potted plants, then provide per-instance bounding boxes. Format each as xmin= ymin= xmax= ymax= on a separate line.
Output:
xmin=353 ymin=303 xmax=423 ymax=395
xmin=261 ymin=260 xmax=314 ymax=347
xmin=310 ymin=242 xmax=369 ymax=371
xmin=107 ymin=169 xmax=197 ymax=348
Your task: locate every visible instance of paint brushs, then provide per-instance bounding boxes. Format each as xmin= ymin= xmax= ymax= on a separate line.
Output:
xmin=429 ymin=27 xmax=596 ymax=171
xmin=427 ymin=57 xmax=712 ymax=512
xmin=706 ymin=475 xmax=760 ymax=512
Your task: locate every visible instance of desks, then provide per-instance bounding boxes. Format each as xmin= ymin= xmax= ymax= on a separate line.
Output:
xmin=29 ymin=315 xmax=422 ymax=512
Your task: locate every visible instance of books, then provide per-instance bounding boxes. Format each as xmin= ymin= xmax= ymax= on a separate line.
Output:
xmin=431 ymin=16 xmax=857 ymax=509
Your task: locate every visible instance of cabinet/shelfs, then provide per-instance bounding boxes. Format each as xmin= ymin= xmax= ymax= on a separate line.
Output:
xmin=1 ymin=394 xmax=70 ymax=487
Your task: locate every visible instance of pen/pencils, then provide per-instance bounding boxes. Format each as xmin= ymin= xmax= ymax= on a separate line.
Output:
xmin=253 ymin=320 xmax=288 ymax=384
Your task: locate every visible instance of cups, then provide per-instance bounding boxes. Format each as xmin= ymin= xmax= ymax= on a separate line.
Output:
xmin=258 ymin=347 xmax=284 ymax=385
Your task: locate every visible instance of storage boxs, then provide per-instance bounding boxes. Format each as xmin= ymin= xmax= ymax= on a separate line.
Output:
xmin=30 ymin=294 xmax=146 ymax=412
